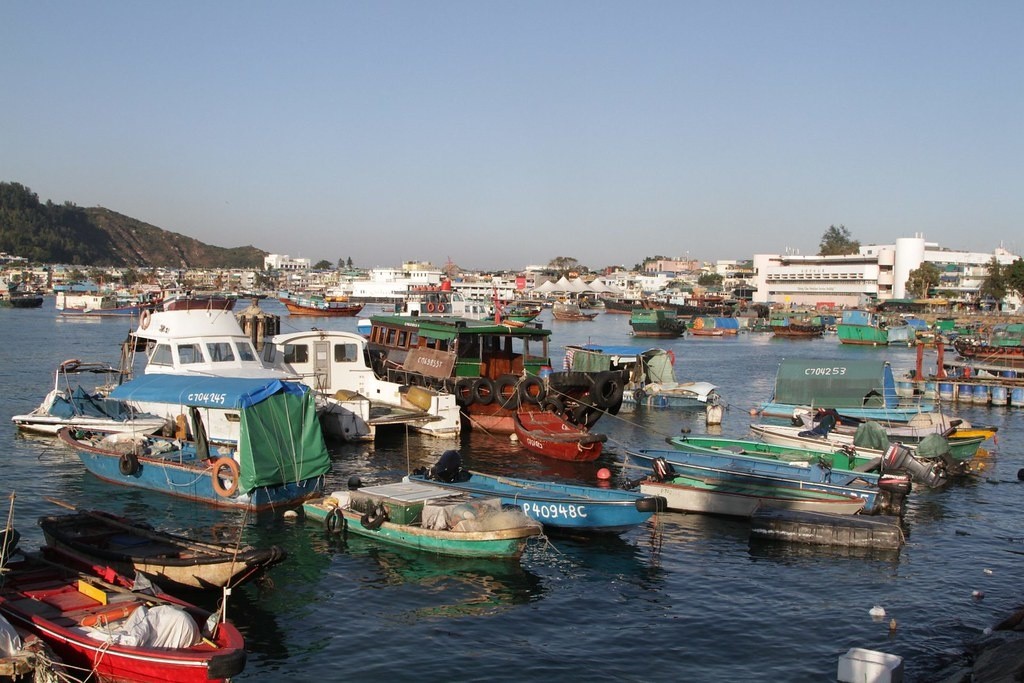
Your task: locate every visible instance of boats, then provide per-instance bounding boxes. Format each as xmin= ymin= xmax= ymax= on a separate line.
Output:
xmin=56 ymin=372 xmax=337 ymax=512
xmin=0 ymin=613 xmax=71 ymax=683
xmin=0 ymin=526 xmax=246 ymax=683
xmin=10 ymin=359 xmax=169 ymax=440
xmin=0 ymin=271 xmax=1024 ymax=519
xmin=36 ymin=494 xmax=286 ymax=615
xmin=300 ymin=480 xmax=546 ymax=561
xmin=404 ymin=450 xmax=667 ymax=542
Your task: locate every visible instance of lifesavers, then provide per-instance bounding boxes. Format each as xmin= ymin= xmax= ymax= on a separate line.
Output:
xmin=455 ymin=377 xmax=495 ymax=406
xmin=635 ymin=496 xmax=667 ymax=513
xmin=954 ymin=341 xmax=1024 ymax=355
xmin=324 ymin=508 xmax=343 ymax=533
xmin=791 ymin=324 xmax=823 ymax=332
xmin=360 ymin=507 xmax=386 ymax=530
xmin=521 ymin=376 xmax=546 ymax=404
xmin=438 ymin=304 xmax=445 ymax=313
xmin=80 ymin=602 xmax=142 ymax=627
xmin=634 ymin=388 xmax=645 ymax=400
xmin=736 ymin=307 xmax=747 ymax=316
xmin=575 ymin=396 xmax=605 ymax=425
xmin=119 ymin=454 xmax=137 ymax=475
xmin=667 ymin=350 xmax=674 ymax=364
xmin=503 ymin=320 xmax=524 ymax=328
xmin=427 ymin=302 xmax=434 ymax=312
xmin=139 ymin=310 xmax=151 ymax=330
xmin=540 ymin=397 xmax=564 ymax=417
xmin=60 ymin=359 xmax=80 ymax=372
xmin=660 ymin=320 xmax=679 ymax=331
xmin=494 ymin=374 xmax=525 ymax=410
xmin=212 ymin=456 xmax=239 ymax=497
xmin=375 ymin=361 xmax=454 ymax=395
xmin=590 ymin=371 xmax=624 ymax=408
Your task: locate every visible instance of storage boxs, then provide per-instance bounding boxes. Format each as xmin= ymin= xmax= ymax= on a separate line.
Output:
xmin=838 ymin=645 xmax=903 ymax=683
